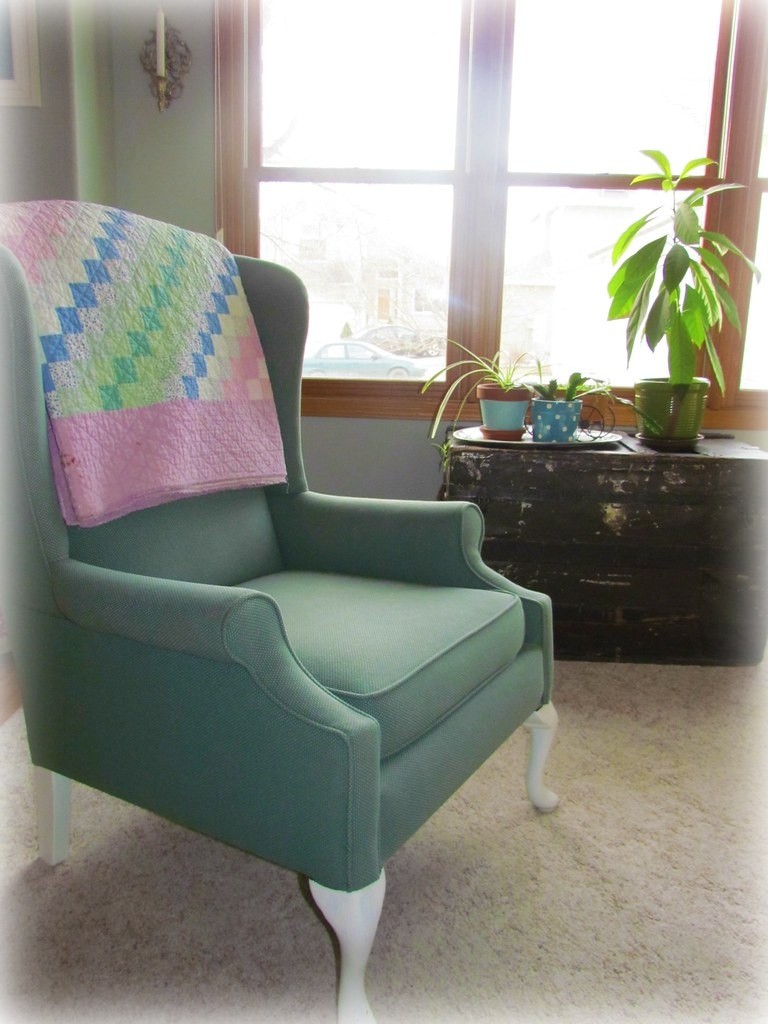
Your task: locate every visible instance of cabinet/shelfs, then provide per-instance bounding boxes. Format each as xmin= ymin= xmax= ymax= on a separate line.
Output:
xmin=443 ymin=426 xmax=768 ymax=664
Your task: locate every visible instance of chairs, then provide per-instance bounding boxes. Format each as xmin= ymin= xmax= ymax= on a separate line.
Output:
xmin=0 ymin=196 xmax=560 ymax=1024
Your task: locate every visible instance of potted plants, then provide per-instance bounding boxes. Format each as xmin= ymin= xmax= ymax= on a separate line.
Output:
xmin=607 ymin=150 xmax=761 ymax=442
xmin=418 ymin=340 xmax=546 ymax=464
xmin=528 ymin=373 xmax=611 ymax=442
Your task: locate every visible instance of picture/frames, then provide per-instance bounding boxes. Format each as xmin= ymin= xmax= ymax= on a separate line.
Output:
xmin=0 ymin=1 xmax=42 ymax=109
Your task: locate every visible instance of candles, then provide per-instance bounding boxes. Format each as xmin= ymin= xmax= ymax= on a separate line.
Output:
xmin=157 ymin=7 xmax=166 ymax=77
